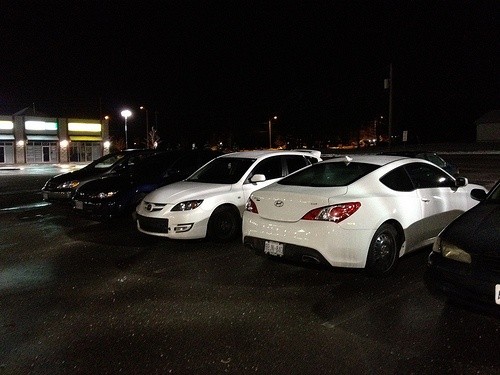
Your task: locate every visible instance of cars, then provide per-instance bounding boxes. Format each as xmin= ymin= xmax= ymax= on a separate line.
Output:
xmin=37 ymin=144 xmax=231 ymax=228
xmin=130 ymin=145 xmax=325 ymax=247
xmin=420 ymin=176 xmax=500 ymax=322
xmin=239 ymin=153 xmax=489 ymax=278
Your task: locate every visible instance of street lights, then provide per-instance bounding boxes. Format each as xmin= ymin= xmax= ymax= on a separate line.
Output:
xmin=139 ymin=106 xmax=149 ymax=149
xmin=121 ymin=109 xmax=132 ymax=149
xmin=268 ymin=115 xmax=278 ymax=150
